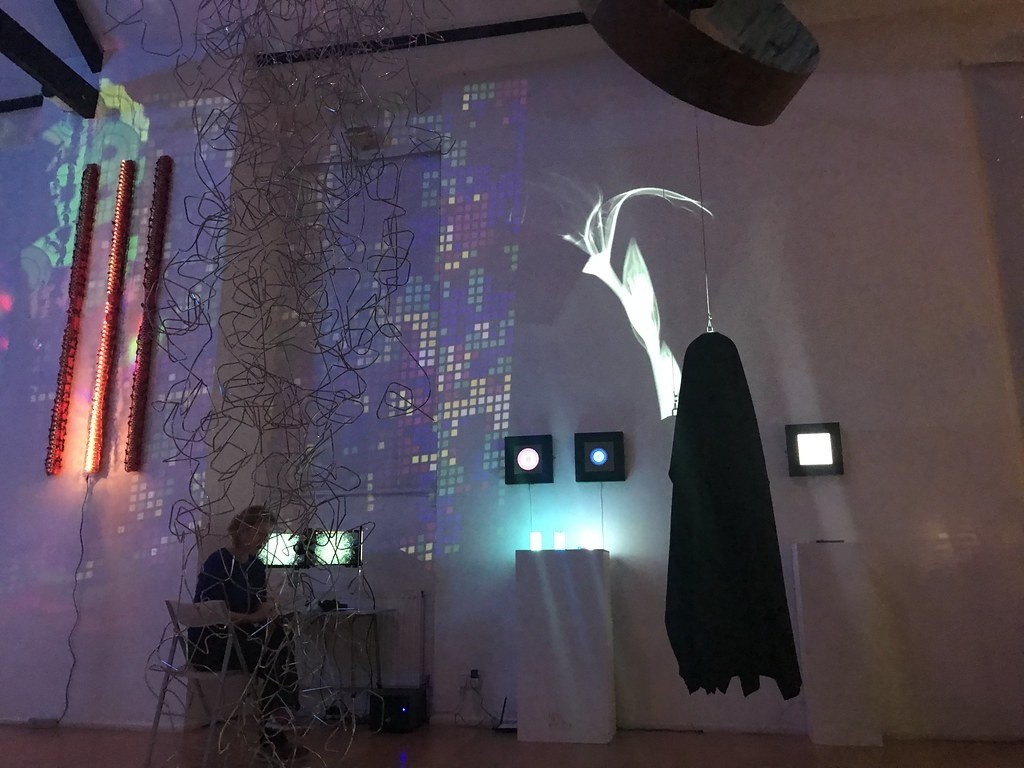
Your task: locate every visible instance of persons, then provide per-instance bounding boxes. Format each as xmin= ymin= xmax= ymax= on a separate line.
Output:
xmin=187 ymin=505 xmax=311 ymax=759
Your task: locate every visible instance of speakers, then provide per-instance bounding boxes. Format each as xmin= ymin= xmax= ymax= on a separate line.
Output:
xmin=577 ymin=0 xmax=820 ymax=125
xmin=369 ymin=686 xmax=428 ymax=732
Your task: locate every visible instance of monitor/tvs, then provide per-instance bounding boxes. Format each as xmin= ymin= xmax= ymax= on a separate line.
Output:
xmin=258 ymin=532 xmax=307 ymax=570
xmin=307 ymin=526 xmax=364 ymax=568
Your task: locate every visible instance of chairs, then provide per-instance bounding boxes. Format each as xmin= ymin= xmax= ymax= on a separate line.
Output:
xmin=141 ymin=600 xmax=287 ymax=768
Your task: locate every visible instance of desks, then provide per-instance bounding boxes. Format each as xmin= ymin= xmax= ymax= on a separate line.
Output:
xmin=276 ymin=601 xmax=397 ymax=725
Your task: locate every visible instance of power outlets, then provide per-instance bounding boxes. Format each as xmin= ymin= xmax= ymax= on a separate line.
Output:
xmin=465 ymin=677 xmax=480 ymax=689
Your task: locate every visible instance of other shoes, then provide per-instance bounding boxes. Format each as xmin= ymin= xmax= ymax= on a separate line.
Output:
xmin=257 ymin=742 xmax=311 ymax=764
